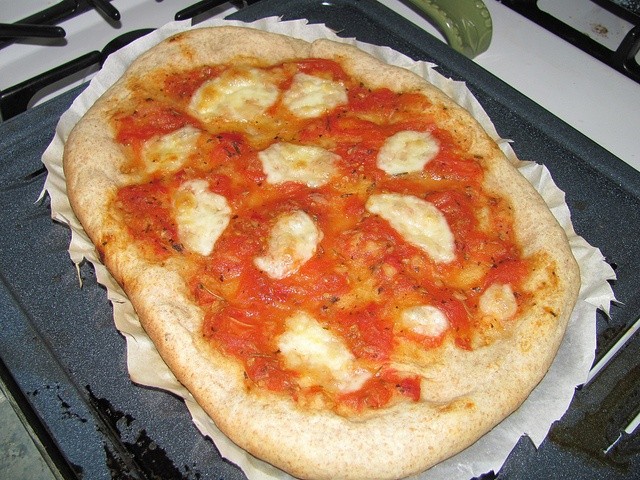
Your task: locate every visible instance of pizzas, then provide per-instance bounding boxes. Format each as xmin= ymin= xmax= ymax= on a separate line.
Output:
xmin=62 ymin=25 xmax=580 ymax=479
